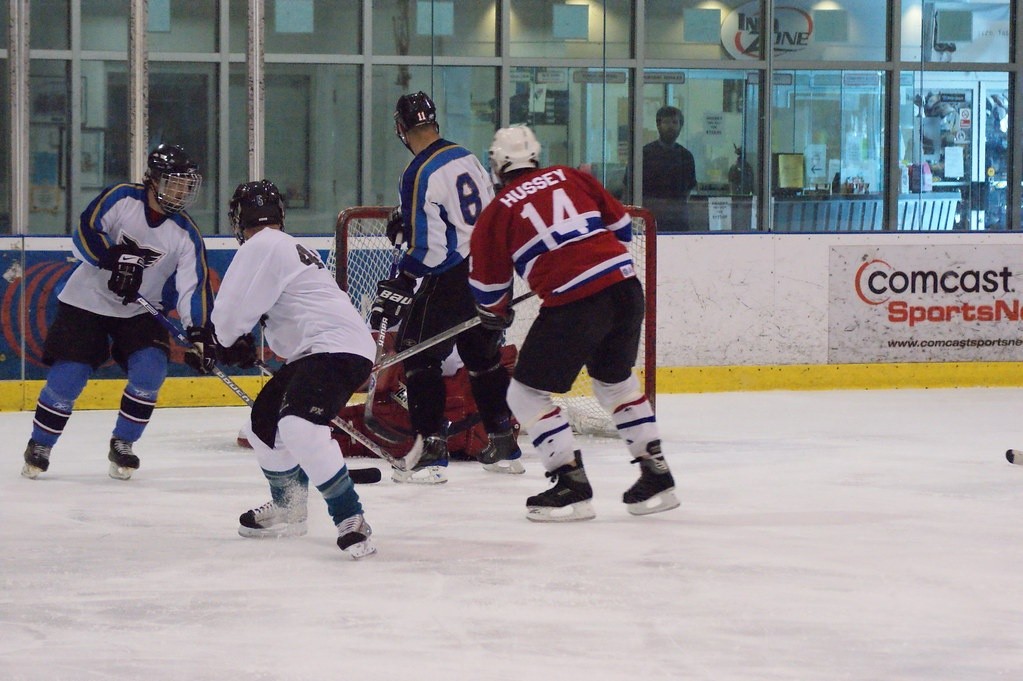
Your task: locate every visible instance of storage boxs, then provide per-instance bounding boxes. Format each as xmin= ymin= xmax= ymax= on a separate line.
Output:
xmin=899 ymin=168 xmax=910 ymax=194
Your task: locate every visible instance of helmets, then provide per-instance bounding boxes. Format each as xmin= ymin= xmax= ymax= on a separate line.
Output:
xmin=394 ymin=91 xmax=437 ymax=135
xmin=142 ymin=143 xmax=198 ymax=185
xmin=229 ymin=179 xmax=285 ymax=245
xmin=487 ymin=125 xmax=541 ymax=173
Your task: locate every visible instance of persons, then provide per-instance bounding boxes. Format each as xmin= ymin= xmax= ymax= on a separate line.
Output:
xmin=623 ymin=106 xmax=701 ymax=232
xmin=237 ymin=92 xmax=526 ymax=483
xmin=203 ymin=180 xmax=377 ymax=559
xmin=468 ymin=125 xmax=680 ymax=522
xmin=21 ymin=144 xmax=211 ymax=481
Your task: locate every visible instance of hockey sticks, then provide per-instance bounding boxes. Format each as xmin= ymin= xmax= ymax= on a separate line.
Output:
xmin=391 ymin=380 xmax=482 ymax=436
xmin=364 ymin=315 xmax=412 ymax=443
xmin=135 ymin=293 xmax=382 ymax=484
xmin=371 ymin=290 xmax=536 ymax=376
xmin=254 ymin=358 xmax=425 ymax=473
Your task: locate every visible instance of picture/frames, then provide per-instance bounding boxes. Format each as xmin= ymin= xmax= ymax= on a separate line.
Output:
xmin=29 ymin=75 xmax=88 ymax=125
xmin=59 ymin=127 xmax=107 ymax=190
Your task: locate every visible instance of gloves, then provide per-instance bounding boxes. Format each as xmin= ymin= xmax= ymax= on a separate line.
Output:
xmin=371 ymin=278 xmax=414 ymax=331
xmin=181 ymin=325 xmax=217 ymax=375
xmin=386 ymin=204 xmax=406 ymax=246
xmin=474 ymin=303 xmax=516 ymax=361
xmin=210 ymin=333 xmax=260 ymax=370
xmin=99 ymin=242 xmax=146 ymax=305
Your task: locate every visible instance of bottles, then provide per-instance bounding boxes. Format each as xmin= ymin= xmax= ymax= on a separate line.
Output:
xmin=845 ymin=175 xmax=870 ymax=194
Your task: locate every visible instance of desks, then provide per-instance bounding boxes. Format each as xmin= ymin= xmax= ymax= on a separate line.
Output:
xmin=932 ymin=181 xmax=968 ymax=187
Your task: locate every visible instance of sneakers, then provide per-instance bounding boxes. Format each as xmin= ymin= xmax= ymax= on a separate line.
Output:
xmin=526 ymin=450 xmax=596 ymax=521
xmin=474 ymin=411 xmax=526 ymax=474
xmin=238 ymin=469 xmax=308 ymax=538
xmin=20 ymin=438 xmax=52 ymax=478
xmin=391 ymin=417 xmax=452 ymax=486
xmin=623 ymin=438 xmax=679 ymax=515
xmin=108 ymin=433 xmax=139 ymax=481
xmin=335 ymin=513 xmax=375 ymax=559
